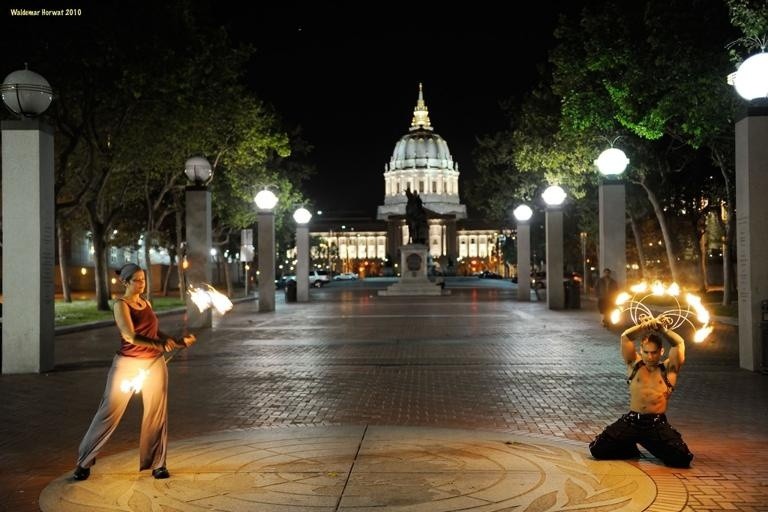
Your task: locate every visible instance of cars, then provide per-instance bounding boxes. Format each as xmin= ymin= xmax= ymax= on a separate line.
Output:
xmin=530 ymin=273 xmax=584 ymax=289
xmin=512 ymin=272 xmax=516 ymax=283
xmin=478 ymin=270 xmax=503 ymax=279
xmin=333 ymin=272 xmax=359 ymax=282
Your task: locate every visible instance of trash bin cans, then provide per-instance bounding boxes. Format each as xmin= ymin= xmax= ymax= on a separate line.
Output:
xmin=284 ymin=279 xmax=297 ymax=302
xmin=563 ymin=280 xmax=580 ymax=309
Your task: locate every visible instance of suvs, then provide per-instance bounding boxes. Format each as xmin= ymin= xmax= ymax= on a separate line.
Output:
xmin=275 ymin=267 xmax=331 ymax=288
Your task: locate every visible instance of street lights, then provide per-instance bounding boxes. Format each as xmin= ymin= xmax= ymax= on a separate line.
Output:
xmin=592 ymin=147 xmax=631 ymax=306
xmin=725 ymin=52 xmax=766 ymax=375
xmin=181 ymin=155 xmax=216 ymax=330
xmin=0 ymin=61 xmax=64 ymax=375
xmin=511 ymin=203 xmax=532 ymax=301
xmin=252 ymin=190 xmax=280 ymax=314
xmin=292 ymin=206 xmax=312 ymax=305
xmin=342 ymin=225 xmax=354 ymax=273
xmin=541 ymin=184 xmax=567 ymax=307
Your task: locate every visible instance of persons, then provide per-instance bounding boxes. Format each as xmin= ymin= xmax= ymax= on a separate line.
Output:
xmin=595 ymin=267 xmax=618 ymax=326
xmin=589 ymin=313 xmax=694 ymax=471
xmin=69 ymin=263 xmax=196 ymax=482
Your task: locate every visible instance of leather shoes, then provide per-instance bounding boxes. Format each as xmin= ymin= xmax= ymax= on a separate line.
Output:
xmin=152 ymin=467 xmax=169 ymax=479
xmin=72 ymin=457 xmax=95 ymax=481
